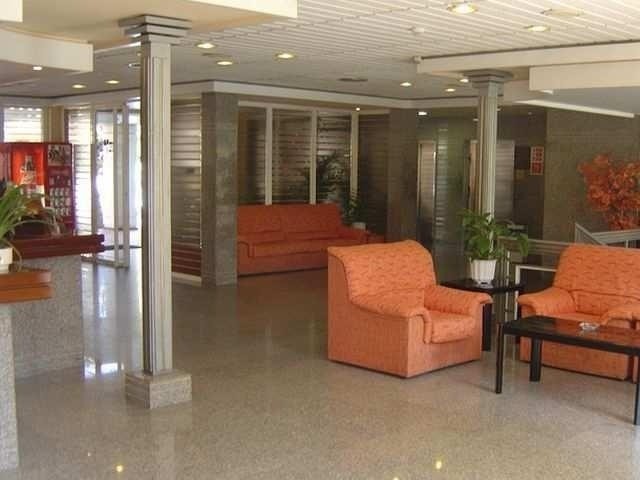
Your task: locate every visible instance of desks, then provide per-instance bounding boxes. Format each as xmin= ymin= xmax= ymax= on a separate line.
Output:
xmin=13 ymin=235 xmax=104 ymax=266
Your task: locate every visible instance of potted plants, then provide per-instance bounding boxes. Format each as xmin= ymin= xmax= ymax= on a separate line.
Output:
xmin=343 ymin=197 xmax=367 ymax=229
xmin=0 ymin=183 xmax=66 ymax=274
xmin=455 ymin=208 xmax=530 ymax=283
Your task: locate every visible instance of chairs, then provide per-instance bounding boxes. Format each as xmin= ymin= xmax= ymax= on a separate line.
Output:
xmin=517 ymin=243 xmax=640 ymax=384
xmin=328 ymin=240 xmax=493 ymax=379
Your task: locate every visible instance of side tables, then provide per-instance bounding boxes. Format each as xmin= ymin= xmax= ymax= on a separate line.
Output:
xmin=441 ymin=278 xmax=526 ymax=351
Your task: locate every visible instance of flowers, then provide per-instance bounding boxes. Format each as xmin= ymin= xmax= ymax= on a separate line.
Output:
xmin=577 ymin=152 xmax=640 ymax=230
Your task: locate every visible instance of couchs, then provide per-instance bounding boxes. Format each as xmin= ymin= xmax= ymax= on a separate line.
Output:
xmin=237 ymin=204 xmax=384 ymax=275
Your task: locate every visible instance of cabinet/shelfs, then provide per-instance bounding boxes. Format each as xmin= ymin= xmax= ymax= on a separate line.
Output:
xmin=0 ymin=142 xmax=74 ymax=230
